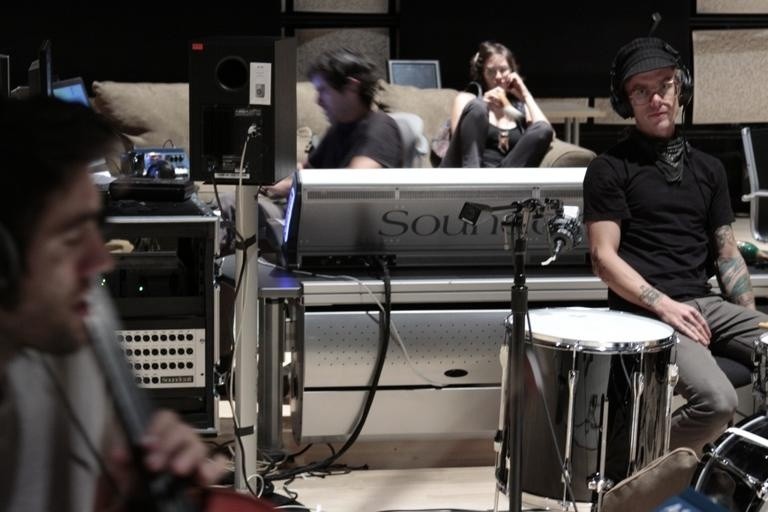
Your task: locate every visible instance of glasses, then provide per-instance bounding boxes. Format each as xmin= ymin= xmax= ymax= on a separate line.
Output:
xmin=628 ymin=78 xmax=676 ymax=105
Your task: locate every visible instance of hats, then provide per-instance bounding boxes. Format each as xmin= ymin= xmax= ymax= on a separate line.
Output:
xmin=614 ymin=37 xmax=676 ymax=89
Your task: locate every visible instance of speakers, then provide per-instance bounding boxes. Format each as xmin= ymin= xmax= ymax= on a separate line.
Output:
xmin=189 ymin=36 xmax=297 ymax=188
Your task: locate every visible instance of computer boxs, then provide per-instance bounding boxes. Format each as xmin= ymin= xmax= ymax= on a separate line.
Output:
xmin=99 ymin=195 xmax=220 ymax=439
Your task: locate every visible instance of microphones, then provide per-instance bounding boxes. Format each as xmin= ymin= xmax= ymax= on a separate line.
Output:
xmin=548 ymin=206 xmax=584 ymax=250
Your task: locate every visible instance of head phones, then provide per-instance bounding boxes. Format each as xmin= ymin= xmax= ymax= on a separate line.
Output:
xmin=469 ymin=51 xmax=518 ymax=87
xmin=608 ymin=43 xmax=694 ymax=121
xmin=122 ymin=150 xmax=175 ymax=178
xmin=0 ymin=225 xmax=21 ymax=312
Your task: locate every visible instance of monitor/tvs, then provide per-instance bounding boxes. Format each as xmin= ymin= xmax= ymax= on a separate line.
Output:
xmin=0 ymin=54 xmax=11 ymax=95
xmin=387 ymin=58 xmax=441 ymax=88
xmin=50 ymin=76 xmax=106 ymax=166
xmin=28 ymin=40 xmax=52 ymax=97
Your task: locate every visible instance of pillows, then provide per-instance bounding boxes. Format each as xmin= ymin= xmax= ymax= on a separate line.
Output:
xmin=94 ymin=80 xmax=196 ymax=166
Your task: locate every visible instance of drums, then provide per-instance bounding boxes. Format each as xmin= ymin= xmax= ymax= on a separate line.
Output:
xmin=689 ymin=414 xmax=767 ymax=512
xmin=493 ymin=307 xmax=678 ymax=512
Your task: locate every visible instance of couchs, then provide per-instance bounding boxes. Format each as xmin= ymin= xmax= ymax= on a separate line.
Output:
xmin=106 ymin=80 xmax=598 ymax=168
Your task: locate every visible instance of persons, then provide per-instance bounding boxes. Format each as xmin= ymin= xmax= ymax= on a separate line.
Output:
xmin=1 ymin=86 xmax=228 ymax=512
xmin=436 ymin=43 xmax=556 ymax=169
xmin=261 ymin=43 xmax=401 ymax=212
xmin=581 ymin=38 xmax=768 ymax=451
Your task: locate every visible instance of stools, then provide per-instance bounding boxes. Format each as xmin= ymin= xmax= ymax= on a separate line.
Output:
xmin=674 ymin=353 xmax=753 ymax=433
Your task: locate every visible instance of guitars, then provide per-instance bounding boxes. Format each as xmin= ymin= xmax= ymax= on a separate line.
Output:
xmin=82 ymin=277 xmax=283 ymax=510
